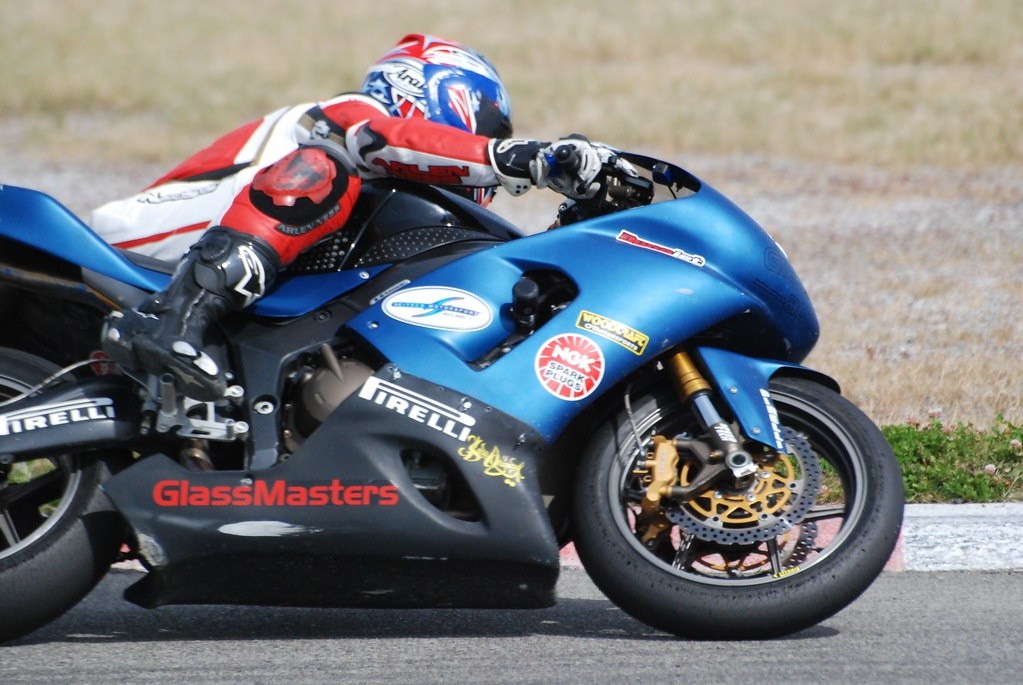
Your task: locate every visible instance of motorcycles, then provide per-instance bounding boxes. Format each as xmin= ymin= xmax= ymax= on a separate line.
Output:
xmin=0 ymin=137 xmax=906 ymax=647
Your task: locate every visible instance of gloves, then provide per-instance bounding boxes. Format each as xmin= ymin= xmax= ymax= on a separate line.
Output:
xmin=489 ymin=137 xmax=639 ymax=215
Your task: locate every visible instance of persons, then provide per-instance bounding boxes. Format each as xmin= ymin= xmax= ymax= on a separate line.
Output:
xmin=88 ymin=36 xmax=639 ymax=404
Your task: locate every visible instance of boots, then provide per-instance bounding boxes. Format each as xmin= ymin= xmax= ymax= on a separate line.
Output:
xmin=102 ymin=225 xmax=284 ymax=402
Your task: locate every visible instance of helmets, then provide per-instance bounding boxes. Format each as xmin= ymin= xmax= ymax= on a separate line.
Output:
xmin=362 ymin=34 xmax=514 ymax=209
